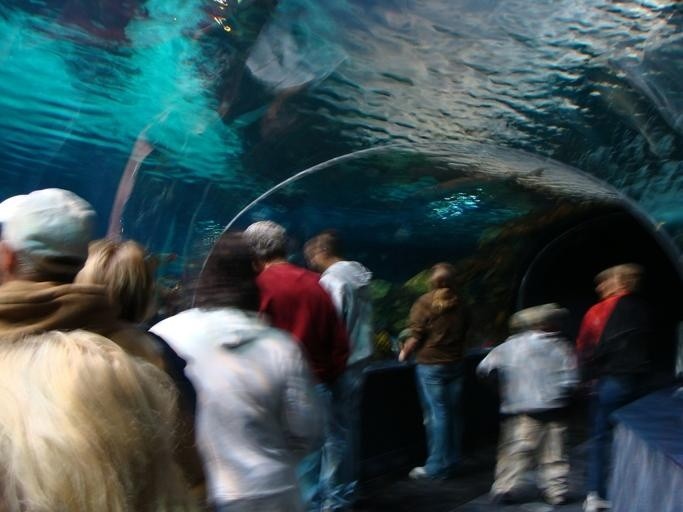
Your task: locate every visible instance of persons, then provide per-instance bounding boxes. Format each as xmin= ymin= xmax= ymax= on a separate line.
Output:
xmin=70 ymin=231 xmax=197 ymax=408
xmin=0 ymin=187 xmax=163 ymax=374
xmin=248 ymin=221 xmax=350 ymax=512
xmin=396 ymin=263 xmax=468 ymax=480
xmin=0 ymin=328 xmax=199 ymax=512
xmin=582 ymin=235 xmax=679 ymax=510
xmin=574 ymin=261 xmax=638 ymax=349
xmin=474 ymin=300 xmax=581 ymax=505
xmin=303 ymin=230 xmax=377 ymax=512
xmin=145 ymin=229 xmax=326 ymax=511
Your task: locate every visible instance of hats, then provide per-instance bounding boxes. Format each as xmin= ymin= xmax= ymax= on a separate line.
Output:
xmin=0 ymin=184 xmax=97 ymax=276
